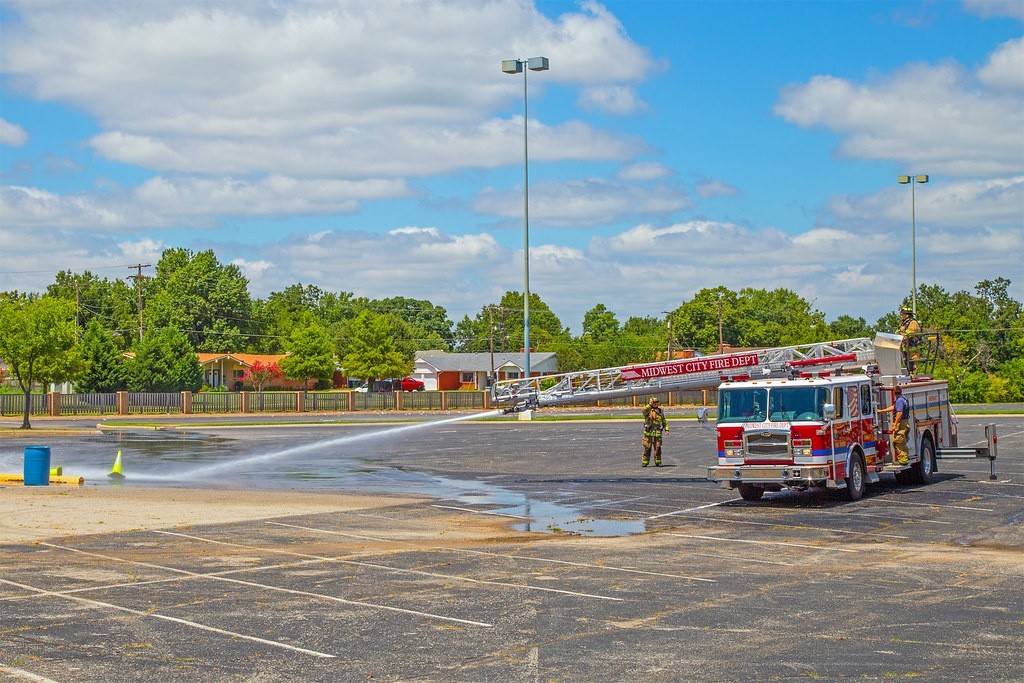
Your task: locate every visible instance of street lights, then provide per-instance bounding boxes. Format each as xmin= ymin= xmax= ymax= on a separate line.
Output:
xmin=502 ymin=56 xmax=549 ymax=382
xmin=897 ymin=175 xmax=928 ymax=320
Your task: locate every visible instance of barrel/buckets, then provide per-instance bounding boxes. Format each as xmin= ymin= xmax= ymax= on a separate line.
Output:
xmin=24 ymin=446 xmax=51 ymax=486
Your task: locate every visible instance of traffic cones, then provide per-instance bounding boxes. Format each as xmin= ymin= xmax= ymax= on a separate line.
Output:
xmin=107 ymin=451 xmax=125 ymax=480
xmin=50 ymin=466 xmax=62 ymax=476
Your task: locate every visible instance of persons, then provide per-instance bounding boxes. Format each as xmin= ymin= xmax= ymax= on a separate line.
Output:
xmin=895 ymin=306 xmax=921 ymax=376
xmin=877 ymin=386 xmax=911 ymax=465
xmin=642 ymin=397 xmax=670 ymax=467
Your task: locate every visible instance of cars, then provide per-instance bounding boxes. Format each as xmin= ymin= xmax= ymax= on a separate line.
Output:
xmin=497 ymin=380 xmax=519 ymax=388
xmin=354 ymin=383 xmax=369 ymax=393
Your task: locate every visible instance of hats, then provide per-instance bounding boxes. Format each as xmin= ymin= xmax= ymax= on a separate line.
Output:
xmin=650 ymin=397 xmax=658 ymax=402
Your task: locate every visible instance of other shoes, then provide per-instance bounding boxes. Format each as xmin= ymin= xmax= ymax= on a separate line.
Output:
xmin=654 ymin=463 xmax=663 ymax=467
xmin=642 ymin=464 xmax=647 ymax=467
xmin=893 ymin=460 xmax=908 ymax=465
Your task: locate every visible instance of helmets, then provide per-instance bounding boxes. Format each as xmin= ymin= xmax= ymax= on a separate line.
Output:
xmin=752 ymin=401 xmax=760 ymax=410
xmin=901 ymin=306 xmax=912 ymax=314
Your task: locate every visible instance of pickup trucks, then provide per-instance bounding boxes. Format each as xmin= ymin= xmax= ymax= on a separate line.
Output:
xmin=392 ymin=376 xmax=424 ymax=392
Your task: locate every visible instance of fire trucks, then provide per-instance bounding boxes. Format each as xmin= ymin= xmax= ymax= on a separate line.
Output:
xmin=491 ymin=331 xmax=1003 ymax=502
